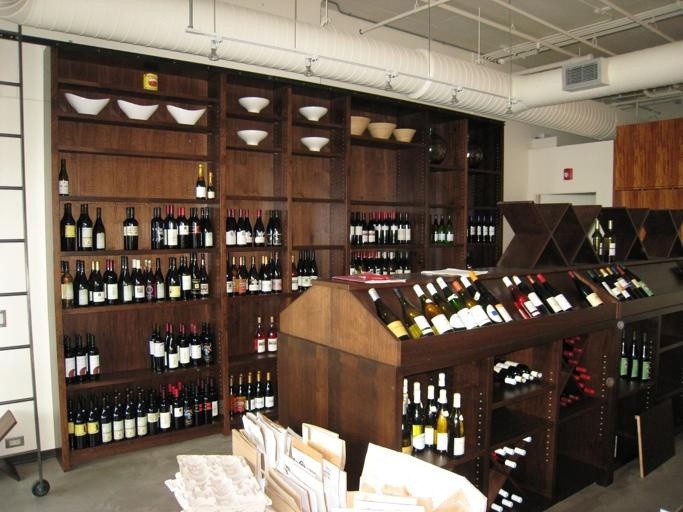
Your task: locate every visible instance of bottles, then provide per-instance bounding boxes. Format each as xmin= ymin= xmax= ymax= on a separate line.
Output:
xmin=194 ymin=163 xmax=206 ymax=198
xmin=224 ymin=208 xmax=283 ymax=296
xmin=603 ymin=219 xmax=616 ymax=262
xmin=148 ymin=319 xmax=212 ymax=374
xmin=367 ymin=263 xmax=653 ymax=340
xmin=429 ymin=215 xmax=495 ymax=245
xmin=206 ymin=172 xmax=216 ymax=199
xmin=591 ymin=219 xmax=604 ymax=262
xmin=229 ymin=370 xmax=274 ymax=418
xmin=57 ymin=202 xmax=214 ymax=310
xmin=264 ymin=315 xmax=277 ymax=352
xmin=57 ymin=157 xmax=70 ymax=196
xmin=290 ymin=249 xmax=323 ymax=292
xmin=561 ymin=335 xmax=596 ymax=408
xmin=347 ymin=211 xmax=412 ymax=276
xmin=401 ymin=372 xmax=466 ymax=458
xmin=492 ymin=358 xmax=545 ymax=387
xmin=620 ymin=326 xmax=654 ymax=379
xmin=487 ymin=436 xmax=532 ymax=512
xmin=64 ymin=332 xmax=100 ymax=384
xmin=252 ymin=315 xmax=265 ymax=354
xmin=68 ymin=375 xmax=219 ymax=450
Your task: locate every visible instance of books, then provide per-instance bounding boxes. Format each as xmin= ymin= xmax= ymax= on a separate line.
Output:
xmin=164 ymin=410 xmax=488 ymax=512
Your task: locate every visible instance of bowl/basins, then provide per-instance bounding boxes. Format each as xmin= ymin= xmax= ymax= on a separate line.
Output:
xmin=63 ymin=92 xmax=110 ymax=116
xmin=298 ymin=105 xmax=328 ymax=122
xmin=115 ymin=99 xmax=159 ymax=121
xmin=351 ymin=116 xmax=416 ymax=144
xmin=164 ymin=105 xmax=206 ymax=127
xmin=301 ymin=137 xmax=330 ymax=152
xmin=234 ymin=129 xmax=267 ymax=146
xmin=235 ymin=97 xmax=271 ymax=115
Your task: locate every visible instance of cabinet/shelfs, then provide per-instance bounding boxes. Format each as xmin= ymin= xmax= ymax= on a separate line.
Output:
xmin=219 ymin=66 xmax=347 ymax=437
xmin=348 ymin=89 xmax=427 ymax=275
xmin=616 ymin=309 xmax=660 ymax=477
xmin=426 ymin=103 xmax=467 ymax=271
xmin=613 ymin=115 xmax=683 ymax=211
xmin=554 ymin=317 xmax=618 ymax=511
xmin=661 ymin=302 xmax=683 ymax=467
xmin=488 ymin=333 xmax=561 ymax=512
xmin=391 ymin=338 xmax=488 ymax=512
xmin=468 ymin=109 xmax=506 ymax=270
xmin=52 ymin=54 xmax=218 ymax=478
